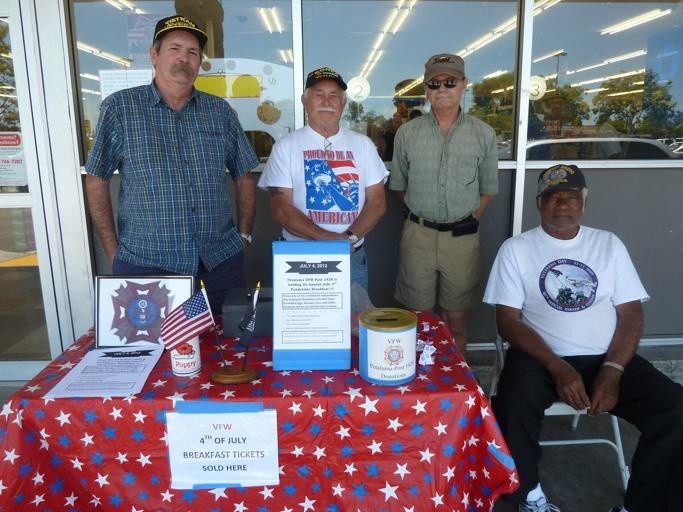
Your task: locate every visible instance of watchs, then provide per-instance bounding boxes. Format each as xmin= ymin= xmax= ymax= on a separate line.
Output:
xmin=241 ymin=232 xmax=252 ymax=243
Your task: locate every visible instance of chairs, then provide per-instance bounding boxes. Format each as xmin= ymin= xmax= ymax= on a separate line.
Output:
xmin=489 ymin=308 xmax=631 ymax=491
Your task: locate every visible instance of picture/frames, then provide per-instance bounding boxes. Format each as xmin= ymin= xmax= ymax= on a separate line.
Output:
xmin=94 ymin=275 xmax=194 ymax=348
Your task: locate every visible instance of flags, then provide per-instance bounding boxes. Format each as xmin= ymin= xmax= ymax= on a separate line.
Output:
xmin=159 ymin=290 xmax=215 ymax=350
xmin=238 ymin=292 xmax=260 ymax=346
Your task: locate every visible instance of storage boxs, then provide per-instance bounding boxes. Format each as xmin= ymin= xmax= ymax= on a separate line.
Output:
xmin=222 ymin=287 xmax=273 ymax=336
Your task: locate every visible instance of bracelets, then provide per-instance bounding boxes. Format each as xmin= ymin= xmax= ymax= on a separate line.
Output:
xmin=344 ymin=230 xmax=358 ymax=241
xmin=602 ymin=360 xmax=625 ymax=373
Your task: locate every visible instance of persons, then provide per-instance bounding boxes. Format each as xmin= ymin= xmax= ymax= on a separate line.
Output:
xmin=257 ymin=65 xmax=391 ymax=316
xmin=83 ymin=15 xmax=262 ymax=325
xmin=378 ymin=79 xmax=427 ymax=161
xmin=482 ymin=165 xmax=683 ymax=512
xmin=529 ymin=102 xmax=551 ymax=158
xmin=590 ymin=100 xmax=624 ymax=160
xmin=390 ymin=54 xmax=500 ymax=354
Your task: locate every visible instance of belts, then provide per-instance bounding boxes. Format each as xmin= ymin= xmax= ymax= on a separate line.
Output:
xmin=401 ymin=205 xmax=453 ymax=231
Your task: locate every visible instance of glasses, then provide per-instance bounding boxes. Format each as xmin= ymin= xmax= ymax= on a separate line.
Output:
xmin=428 ymin=77 xmax=458 ymax=89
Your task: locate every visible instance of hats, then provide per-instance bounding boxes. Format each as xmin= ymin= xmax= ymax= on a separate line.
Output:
xmin=535 ymin=165 xmax=587 ymax=198
xmin=423 ymin=54 xmax=464 ymax=86
xmin=306 ymin=67 xmax=347 ymax=90
xmin=154 ymin=14 xmax=208 ymax=50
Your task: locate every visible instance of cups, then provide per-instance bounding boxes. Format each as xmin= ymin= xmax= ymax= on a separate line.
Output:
xmin=169 ymin=331 xmax=201 ymax=376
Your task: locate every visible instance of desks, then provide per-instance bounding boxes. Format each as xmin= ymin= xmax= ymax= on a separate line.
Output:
xmin=0 ymin=312 xmax=521 ymax=512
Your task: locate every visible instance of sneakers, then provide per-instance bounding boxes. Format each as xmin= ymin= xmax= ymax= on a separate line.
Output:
xmin=518 ymin=497 xmax=551 ymax=512
